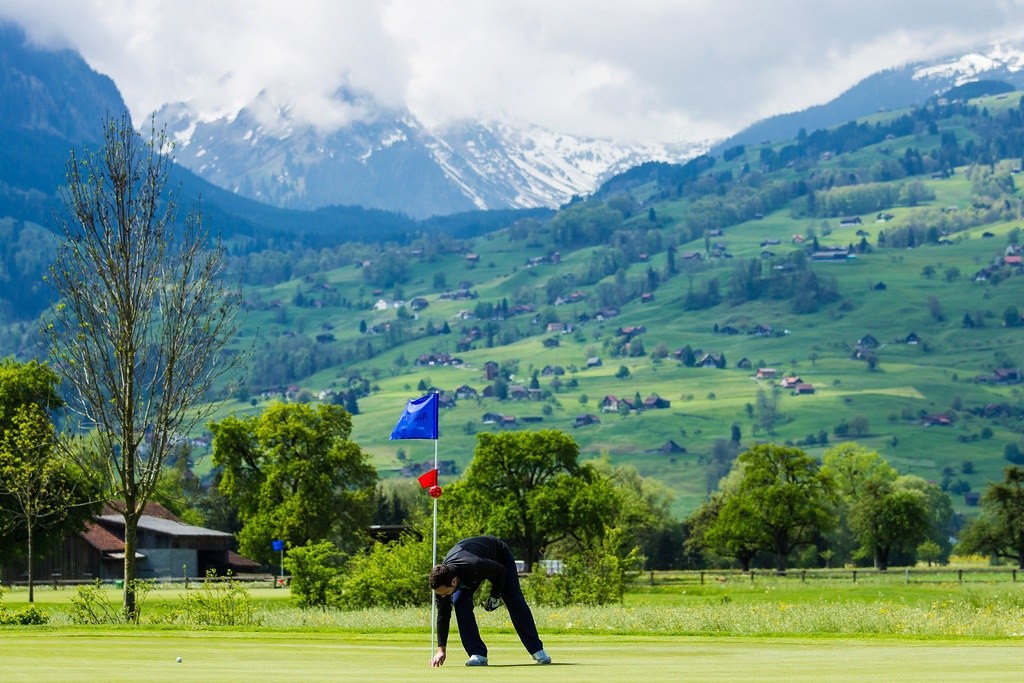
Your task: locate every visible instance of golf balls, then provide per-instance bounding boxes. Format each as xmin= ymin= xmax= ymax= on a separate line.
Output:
xmin=176 ymin=657 xmax=182 ymax=663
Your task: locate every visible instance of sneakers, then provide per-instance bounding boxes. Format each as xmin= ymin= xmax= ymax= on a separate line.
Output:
xmin=465 ymin=655 xmax=488 ymax=666
xmin=532 ymin=650 xmax=552 ymax=664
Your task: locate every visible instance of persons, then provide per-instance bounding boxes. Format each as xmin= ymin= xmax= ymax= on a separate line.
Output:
xmin=429 ymin=536 xmax=551 ymax=667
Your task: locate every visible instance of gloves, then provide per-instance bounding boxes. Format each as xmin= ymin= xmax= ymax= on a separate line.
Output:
xmin=485 ymin=597 xmax=500 ymax=611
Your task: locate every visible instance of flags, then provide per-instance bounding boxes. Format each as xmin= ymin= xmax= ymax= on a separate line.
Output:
xmin=389 ymin=393 xmax=439 ymax=439
xmin=418 ymin=470 xmax=437 ymax=489
xmin=272 ymin=541 xmax=282 ymax=551
